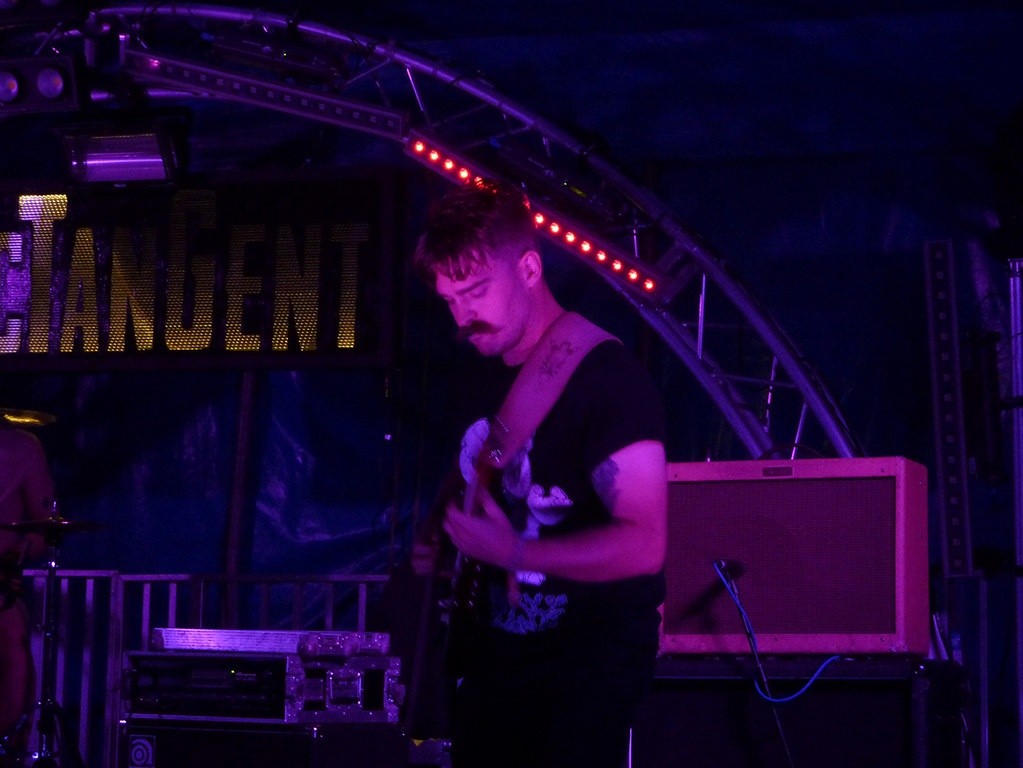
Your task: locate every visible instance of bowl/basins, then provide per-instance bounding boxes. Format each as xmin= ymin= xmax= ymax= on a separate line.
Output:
xmin=32 ymin=497 xmax=71 ymax=521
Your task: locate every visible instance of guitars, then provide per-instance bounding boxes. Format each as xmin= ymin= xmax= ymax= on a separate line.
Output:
xmin=435 ymin=414 xmax=499 ymax=697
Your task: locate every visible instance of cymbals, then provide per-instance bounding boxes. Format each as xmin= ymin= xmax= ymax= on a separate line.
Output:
xmin=0 ymin=514 xmax=108 ymax=536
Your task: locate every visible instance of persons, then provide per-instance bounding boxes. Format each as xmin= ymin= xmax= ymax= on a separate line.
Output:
xmin=390 ymin=179 xmax=668 ymax=768
xmin=0 ymin=384 xmax=61 ymax=754
